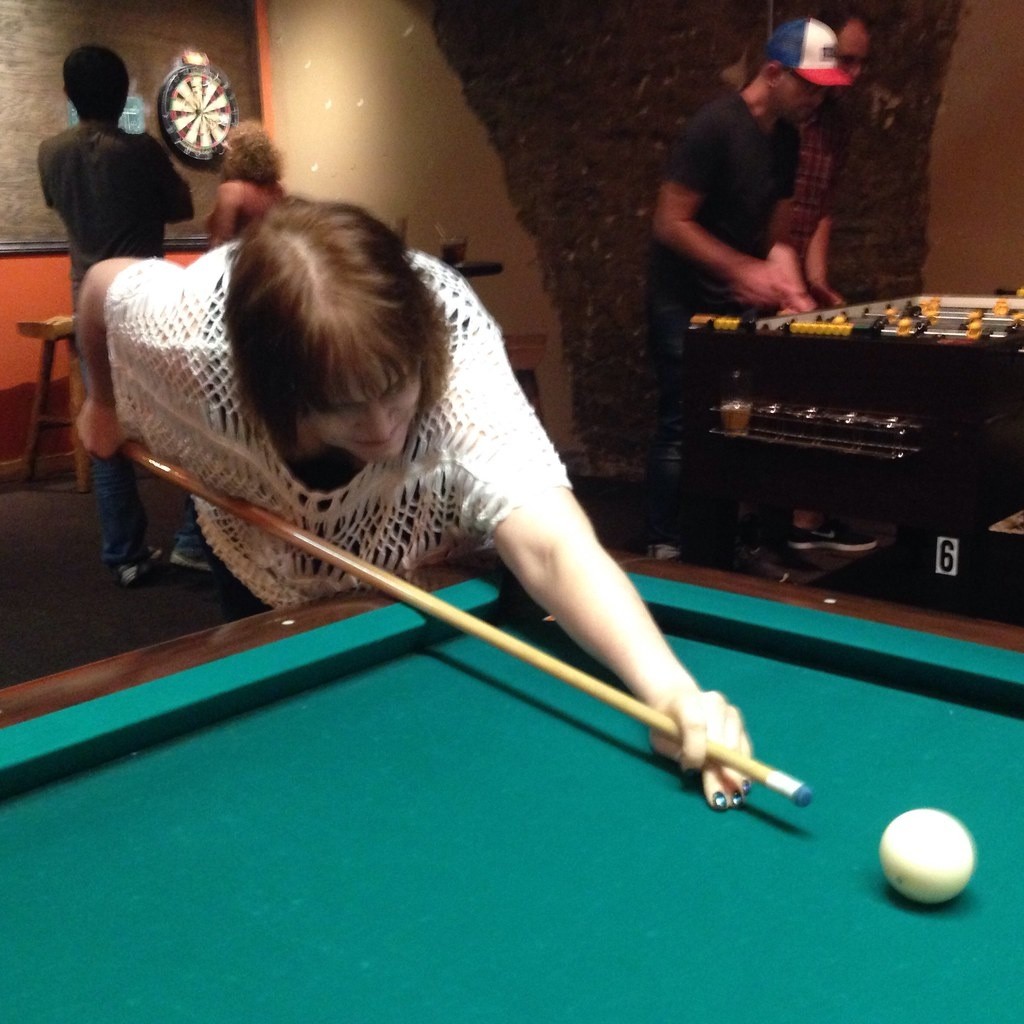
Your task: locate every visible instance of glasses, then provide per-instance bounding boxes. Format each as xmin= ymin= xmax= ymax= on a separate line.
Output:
xmin=835 ymin=53 xmax=871 ymax=75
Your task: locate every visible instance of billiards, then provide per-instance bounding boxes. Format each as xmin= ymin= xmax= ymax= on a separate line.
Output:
xmin=880 ymin=804 xmax=975 ymax=907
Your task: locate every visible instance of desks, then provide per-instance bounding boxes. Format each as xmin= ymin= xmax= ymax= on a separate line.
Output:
xmin=2 ymin=531 xmax=1020 ymax=1015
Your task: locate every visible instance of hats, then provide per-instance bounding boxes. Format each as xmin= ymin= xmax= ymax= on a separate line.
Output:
xmin=765 ymin=17 xmax=853 ymax=87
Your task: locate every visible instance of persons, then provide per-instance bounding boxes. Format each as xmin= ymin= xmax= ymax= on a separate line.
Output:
xmin=77 ymin=200 xmax=754 ymax=812
xmin=755 ymin=12 xmax=880 ymax=553
xmin=647 ymin=14 xmax=852 ymax=561
xmin=205 ymin=119 xmax=285 ymax=251
xmin=38 ymin=45 xmax=212 ymax=587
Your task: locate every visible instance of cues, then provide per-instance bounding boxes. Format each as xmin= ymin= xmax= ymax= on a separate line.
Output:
xmin=115 ymin=431 xmax=817 ymax=811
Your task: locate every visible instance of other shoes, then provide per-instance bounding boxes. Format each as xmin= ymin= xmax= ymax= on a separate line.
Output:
xmin=168 ymin=534 xmax=212 ymax=572
xmin=113 ymin=545 xmax=162 ymax=584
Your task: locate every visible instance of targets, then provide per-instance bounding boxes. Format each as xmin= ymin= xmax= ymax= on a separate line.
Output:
xmin=156 ymin=61 xmax=241 ymax=164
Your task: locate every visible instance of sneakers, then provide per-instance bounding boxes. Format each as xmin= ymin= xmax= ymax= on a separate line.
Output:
xmin=786 ymin=517 xmax=879 ymax=552
xmin=632 ymin=534 xmax=682 ymax=561
xmin=738 ymin=513 xmax=764 ymax=553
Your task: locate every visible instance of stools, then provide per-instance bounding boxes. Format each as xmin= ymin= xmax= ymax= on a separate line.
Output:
xmin=16 ymin=319 xmax=93 ymax=494
